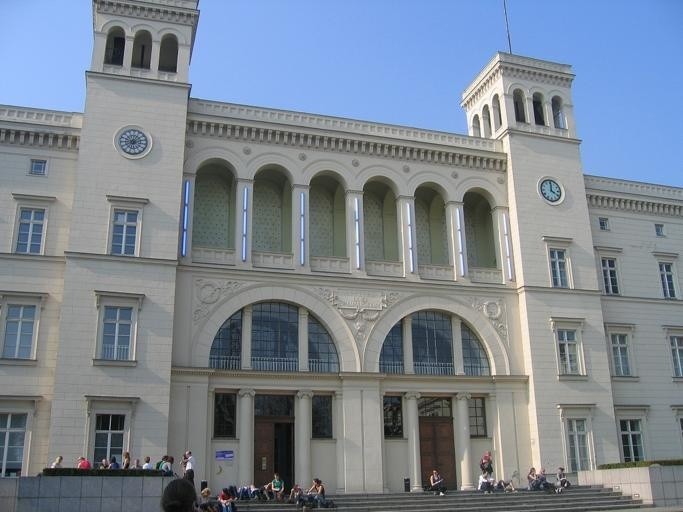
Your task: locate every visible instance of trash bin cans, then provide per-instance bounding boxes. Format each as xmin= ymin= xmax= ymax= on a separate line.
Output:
xmin=404 ymin=478 xmax=410 ymax=492
xmin=201 ymin=480 xmax=207 ymax=492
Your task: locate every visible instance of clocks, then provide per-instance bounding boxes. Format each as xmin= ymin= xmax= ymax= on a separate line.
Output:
xmin=536 ymin=176 xmax=565 ymax=206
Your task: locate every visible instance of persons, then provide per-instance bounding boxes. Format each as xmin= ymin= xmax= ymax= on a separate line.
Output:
xmin=477 ymin=450 xmax=570 ymax=495
xmin=49 ymin=450 xmax=326 ymax=511
xmin=429 ymin=468 xmax=447 ymax=496
